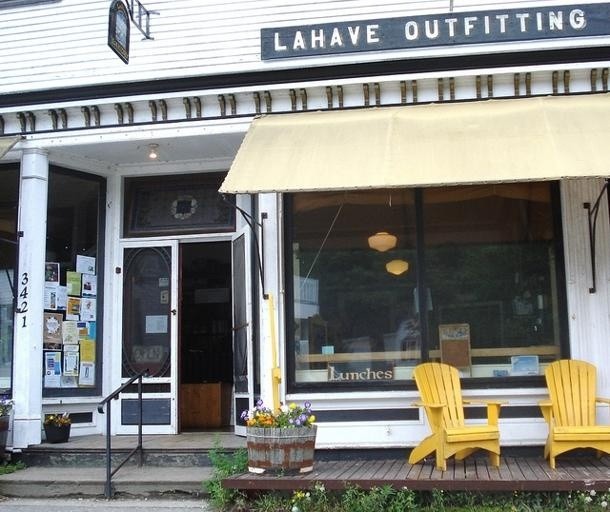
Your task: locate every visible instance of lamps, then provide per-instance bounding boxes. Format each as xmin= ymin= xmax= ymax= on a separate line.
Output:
xmin=366 ymin=230 xmax=410 ymax=275
xmin=148 ymin=143 xmax=159 ymax=159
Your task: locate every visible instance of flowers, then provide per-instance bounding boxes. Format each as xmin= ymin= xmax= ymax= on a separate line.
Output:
xmin=240 ymin=398 xmax=317 ymax=427
xmin=44 ymin=411 xmax=72 ymax=426
xmin=0 ymin=392 xmax=15 ymax=416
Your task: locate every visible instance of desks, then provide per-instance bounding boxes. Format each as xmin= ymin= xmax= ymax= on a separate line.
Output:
xmin=180 ymin=382 xmax=231 ymax=429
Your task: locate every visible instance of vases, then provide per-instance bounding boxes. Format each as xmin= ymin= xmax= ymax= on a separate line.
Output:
xmin=246 ymin=423 xmax=318 ymax=476
xmin=0 ymin=416 xmax=10 ymax=452
xmin=44 ymin=426 xmax=71 ymax=443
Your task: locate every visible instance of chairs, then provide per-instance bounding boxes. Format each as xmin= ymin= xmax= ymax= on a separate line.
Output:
xmin=407 ymin=358 xmax=609 ymax=470
